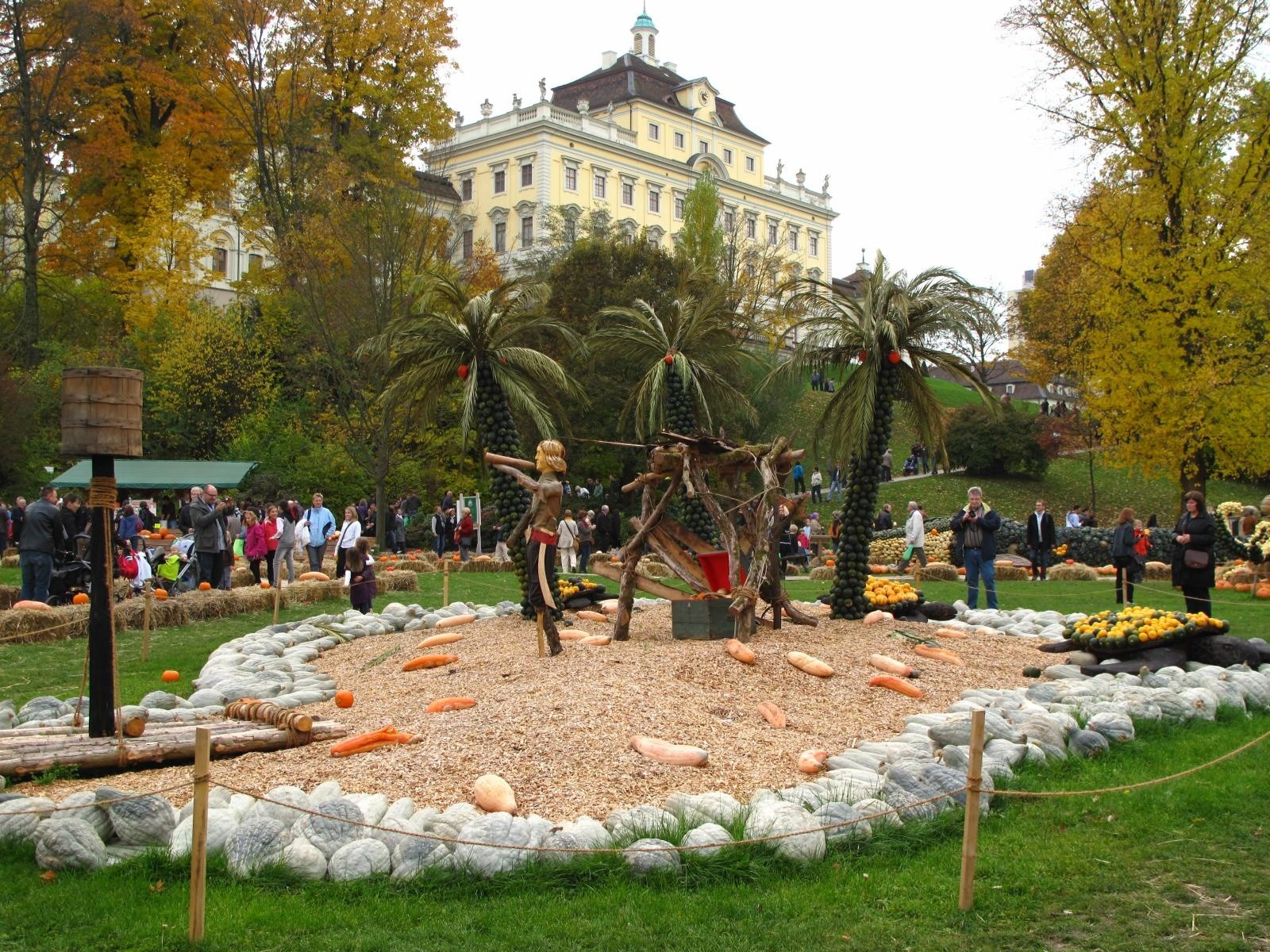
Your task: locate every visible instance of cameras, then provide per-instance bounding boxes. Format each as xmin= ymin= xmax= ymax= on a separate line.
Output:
xmin=223 ymin=496 xmax=228 ymax=503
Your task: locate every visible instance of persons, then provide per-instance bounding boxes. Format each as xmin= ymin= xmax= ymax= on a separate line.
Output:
xmin=891 ymin=501 xmax=928 ymax=576
xmin=431 ymin=504 xmax=446 ymax=559
xmin=1027 ymin=499 xmax=1056 ymax=581
xmin=302 ymin=493 xmax=336 ymax=572
xmin=493 ymin=439 xmax=568 ymax=657
xmin=765 ymin=441 xmax=1270 ymax=618
xmin=811 ymin=368 xmax=836 ymax=393
xmin=0 ymin=484 xmax=474 ymax=617
xmin=1000 ymin=393 xmax=1017 ymax=413
xmin=951 ymin=487 xmax=1001 ymax=610
xmin=1171 ymin=490 xmax=1218 ymax=619
xmin=554 ymin=472 xmax=623 ymax=576
xmin=1037 ymin=397 xmax=1080 ymax=420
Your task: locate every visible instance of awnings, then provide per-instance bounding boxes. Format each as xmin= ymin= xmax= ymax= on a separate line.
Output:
xmin=48 ymin=458 xmax=263 ymax=489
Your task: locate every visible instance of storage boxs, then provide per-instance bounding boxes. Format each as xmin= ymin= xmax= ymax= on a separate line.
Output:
xmin=671 ymin=550 xmax=757 ymax=639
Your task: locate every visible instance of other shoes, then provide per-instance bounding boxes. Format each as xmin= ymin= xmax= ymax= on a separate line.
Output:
xmin=573 ymin=567 xmax=576 ymax=572
xmin=892 ymin=568 xmax=902 ymax=576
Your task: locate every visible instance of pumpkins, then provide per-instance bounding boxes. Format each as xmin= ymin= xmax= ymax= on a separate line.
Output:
xmin=664 ymin=355 xmax=720 ymax=557
xmin=817 ymin=350 xmax=901 ymax=621
xmin=457 ymin=357 xmax=565 ymax=624
xmin=827 ymin=495 xmax=1270 ymax=598
xmin=0 ymin=525 xmax=1270 ymax=876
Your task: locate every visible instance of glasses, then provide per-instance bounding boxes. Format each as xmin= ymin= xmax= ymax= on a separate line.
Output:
xmin=205 ymin=492 xmax=217 ymax=497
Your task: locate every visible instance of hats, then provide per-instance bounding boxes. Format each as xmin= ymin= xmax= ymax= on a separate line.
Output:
xmin=171 ymin=541 xmax=181 ymax=551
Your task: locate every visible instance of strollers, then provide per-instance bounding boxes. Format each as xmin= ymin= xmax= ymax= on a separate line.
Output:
xmin=45 ymin=534 xmax=93 ymax=608
xmin=156 ymin=531 xmax=200 ymax=598
xmin=124 ymin=534 xmax=169 ymax=599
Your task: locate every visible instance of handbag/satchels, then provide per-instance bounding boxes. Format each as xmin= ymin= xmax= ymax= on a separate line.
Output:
xmin=573 ymin=538 xmax=579 ymax=550
xmin=1184 ymin=549 xmax=1209 ymax=569
xmin=233 ymin=538 xmax=244 ymax=557
xmin=462 ymin=537 xmax=470 ymax=546
xmin=902 ymin=544 xmax=914 ymax=561
xmin=137 ymin=517 xmax=142 ymax=532
xmin=118 ymin=549 xmax=139 ymax=577
